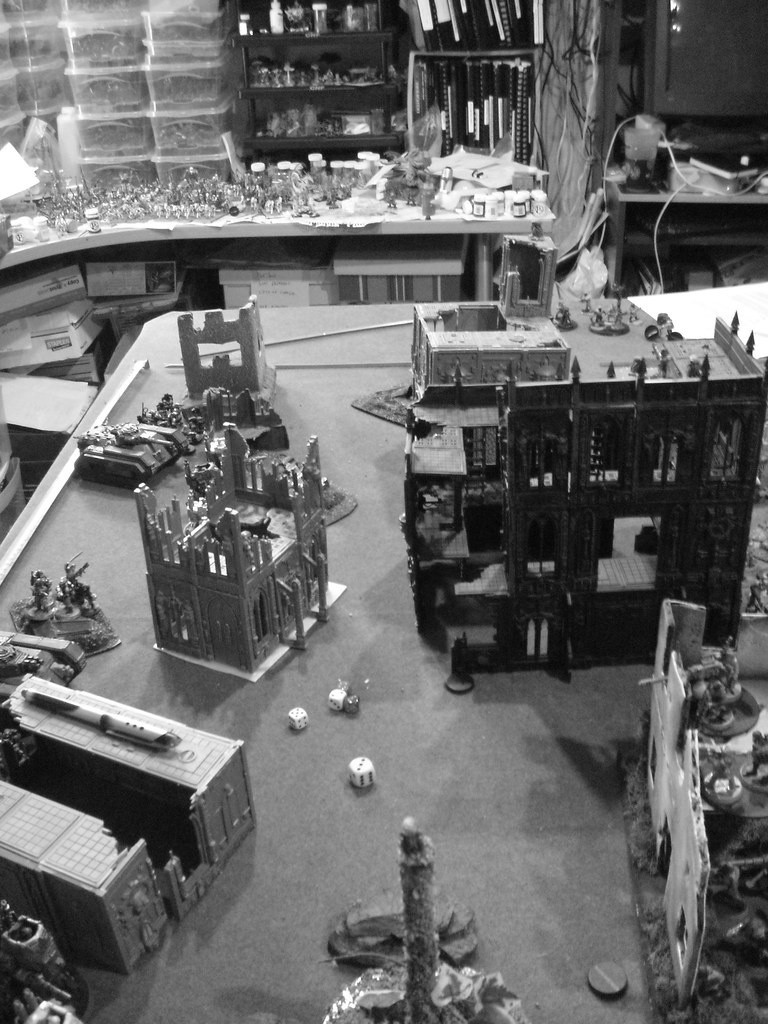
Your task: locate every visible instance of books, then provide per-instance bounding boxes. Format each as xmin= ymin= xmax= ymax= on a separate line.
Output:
xmin=399 ymin=1 xmax=546 ymax=52
xmin=416 ymin=55 xmax=534 ymax=169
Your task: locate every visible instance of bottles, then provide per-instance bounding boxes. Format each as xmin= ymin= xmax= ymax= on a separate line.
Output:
xmin=269 ymin=0 xmax=284 ymax=34
xmin=308 ymin=153 xmax=327 ymax=172
xmin=277 ymin=160 xmax=302 ymax=173
xmin=33 ymin=217 xmax=50 ymax=241
xmin=239 ymin=14 xmax=251 ymax=36
xmin=474 ymin=190 xmax=547 ymax=217
xmin=312 ymin=3 xmax=327 ymax=33
xmin=85 ymin=209 xmax=101 ymax=233
xmin=250 ymin=162 xmax=265 ymax=176
xmin=330 ymin=151 xmax=388 ymax=177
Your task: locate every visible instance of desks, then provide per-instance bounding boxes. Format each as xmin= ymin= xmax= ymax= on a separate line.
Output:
xmin=0 ymin=203 xmax=556 ymax=272
xmin=0 ymin=304 xmax=768 ymax=1024
xmin=594 ymin=148 xmax=768 ymax=295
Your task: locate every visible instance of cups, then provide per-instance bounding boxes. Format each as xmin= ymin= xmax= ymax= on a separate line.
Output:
xmin=624 ymin=128 xmax=659 ymax=193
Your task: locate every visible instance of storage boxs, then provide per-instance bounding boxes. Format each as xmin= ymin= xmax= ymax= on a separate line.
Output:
xmin=333 ymin=234 xmax=471 ymax=303
xmin=0 ymin=297 xmax=108 ymax=369
xmin=0 ymin=263 xmax=87 ymax=326
xmin=0 ymin=0 xmax=244 ymax=191
xmin=218 ymin=266 xmax=334 ymax=307
xmin=87 ymin=258 xmax=179 ymax=297
